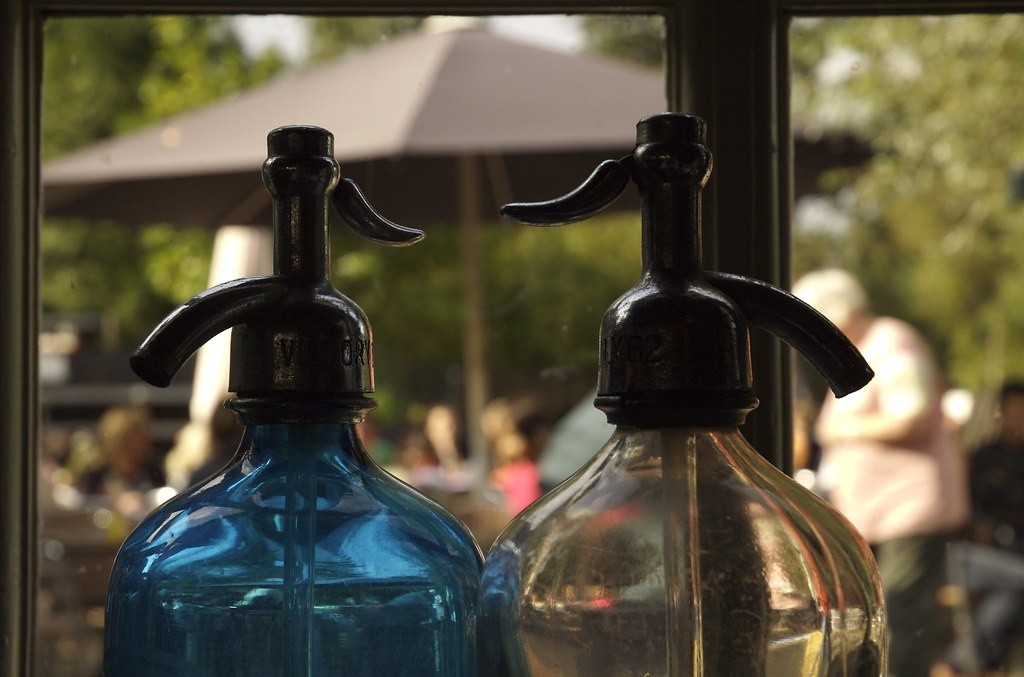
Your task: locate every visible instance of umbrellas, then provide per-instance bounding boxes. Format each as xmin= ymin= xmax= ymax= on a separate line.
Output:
xmin=36 ymin=29 xmax=866 ymax=474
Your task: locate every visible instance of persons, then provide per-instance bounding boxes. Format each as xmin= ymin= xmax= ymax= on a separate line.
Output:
xmin=790 ymin=270 xmax=968 ymax=677
xmin=77 ymin=407 xmax=163 ymax=493
xmin=926 ymin=383 xmax=1023 ymax=677
xmin=355 ymin=388 xmax=613 ymax=517
